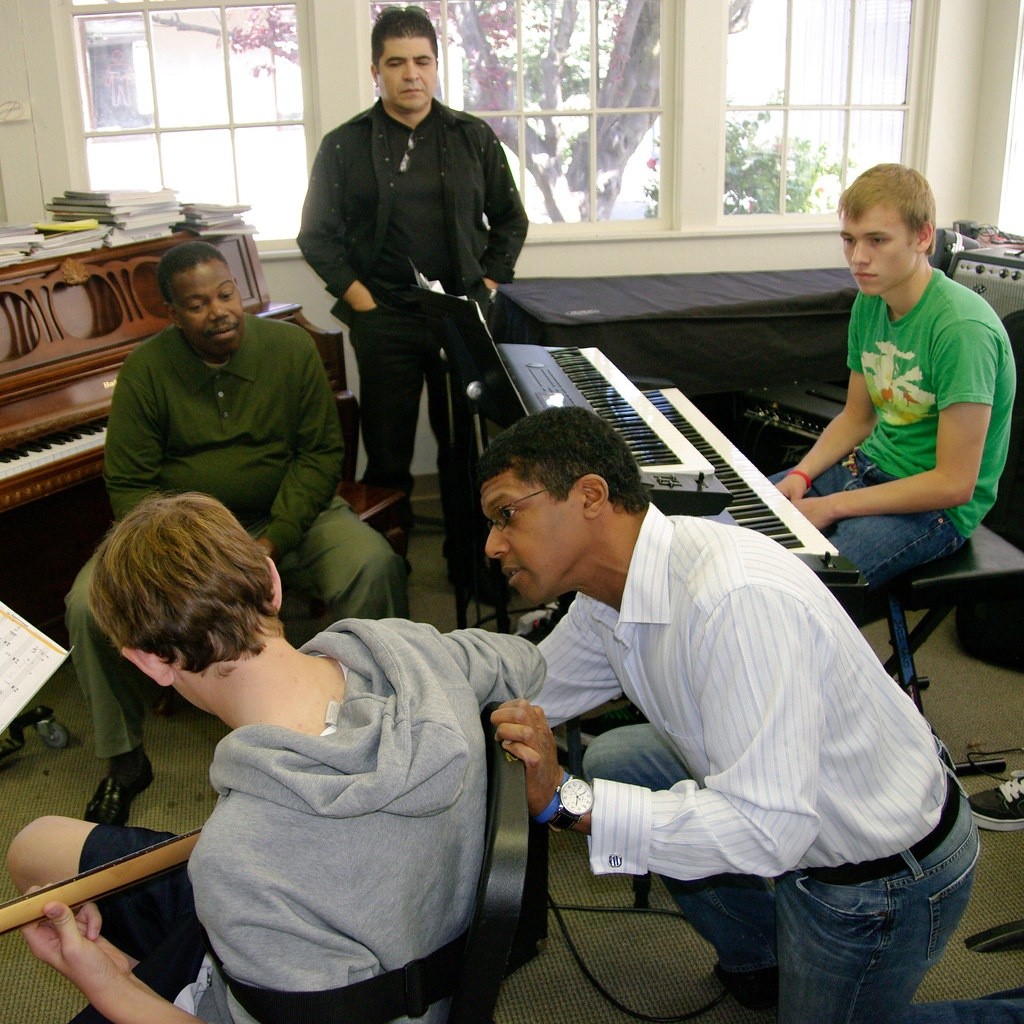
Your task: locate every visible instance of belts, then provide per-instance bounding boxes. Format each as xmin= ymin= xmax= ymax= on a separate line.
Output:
xmin=797 ymin=726 xmax=959 ymax=886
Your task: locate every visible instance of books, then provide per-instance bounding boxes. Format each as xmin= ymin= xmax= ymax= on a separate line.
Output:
xmin=0 ymin=188 xmax=259 ymax=270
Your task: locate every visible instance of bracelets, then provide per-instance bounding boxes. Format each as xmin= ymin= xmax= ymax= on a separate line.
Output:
xmin=535 ymin=771 xmax=569 ymax=824
xmin=785 ymin=469 xmax=811 ymax=493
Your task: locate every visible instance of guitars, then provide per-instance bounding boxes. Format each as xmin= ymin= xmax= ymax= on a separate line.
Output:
xmin=1 ymin=827 xmax=203 ymax=935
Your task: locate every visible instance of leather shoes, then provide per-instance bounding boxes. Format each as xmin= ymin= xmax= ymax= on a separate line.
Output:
xmin=82 ymin=753 xmax=154 ymax=825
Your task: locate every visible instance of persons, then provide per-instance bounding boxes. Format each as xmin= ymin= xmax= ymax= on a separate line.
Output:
xmin=4 ymin=492 xmax=549 ymax=1023
xmin=65 ymin=243 xmax=411 ymax=826
xmin=767 ymin=162 xmax=1016 ymax=588
xmin=295 ymin=7 xmax=528 ymax=601
xmin=468 ymin=405 xmax=1024 ymax=1024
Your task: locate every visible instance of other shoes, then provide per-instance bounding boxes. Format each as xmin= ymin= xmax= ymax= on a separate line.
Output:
xmin=712 ymin=960 xmax=780 ymax=1009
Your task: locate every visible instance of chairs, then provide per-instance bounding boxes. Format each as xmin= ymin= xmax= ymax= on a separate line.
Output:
xmin=69 ymin=701 xmax=551 ymax=1024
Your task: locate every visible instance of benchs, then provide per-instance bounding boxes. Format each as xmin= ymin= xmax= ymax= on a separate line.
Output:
xmin=338 ymin=479 xmax=412 ymax=618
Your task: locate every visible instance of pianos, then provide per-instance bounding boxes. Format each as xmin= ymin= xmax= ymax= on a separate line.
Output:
xmin=0 ymin=226 xmax=364 ymax=625
xmin=494 ymin=340 xmax=871 ymax=908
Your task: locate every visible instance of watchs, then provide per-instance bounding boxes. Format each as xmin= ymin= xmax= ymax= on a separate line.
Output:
xmin=489 ymin=288 xmax=498 ymax=300
xmin=548 ymin=774 xmax=594 ymax=833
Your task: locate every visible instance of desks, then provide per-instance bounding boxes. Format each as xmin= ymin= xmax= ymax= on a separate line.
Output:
xmin=486 ymin=266 xmax=862 ymax=394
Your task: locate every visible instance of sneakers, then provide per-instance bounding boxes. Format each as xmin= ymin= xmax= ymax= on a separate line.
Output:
xmin=965 ymin=769 xmax=1024 ymax=833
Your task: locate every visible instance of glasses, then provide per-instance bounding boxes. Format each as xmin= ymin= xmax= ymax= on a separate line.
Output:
xmin=375 ymin=5 xmax=429 ymax=22
xmin=395 ymin=129 xmax=423 ymax=174
xmin=486 ymin=470 xmax=591 ymax=531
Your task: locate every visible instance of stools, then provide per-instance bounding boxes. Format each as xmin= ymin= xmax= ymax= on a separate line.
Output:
xmin=851 ymin=521 xmax=1024 ymax=778
